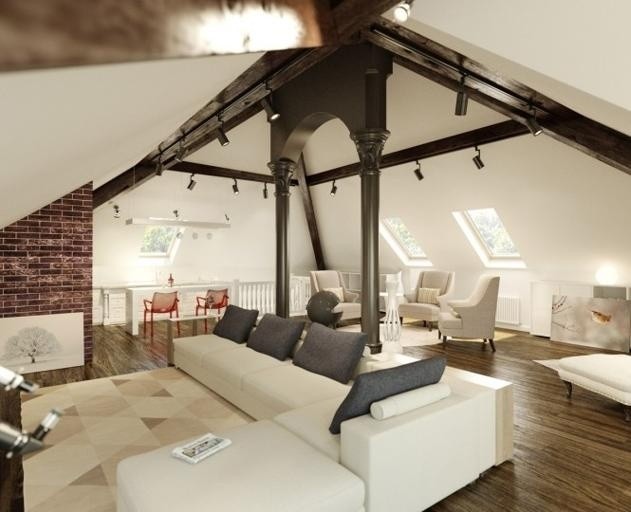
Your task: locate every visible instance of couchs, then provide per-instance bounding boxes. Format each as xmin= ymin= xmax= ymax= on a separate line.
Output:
xmin=174 ymin=317 xmax=515 ymax=512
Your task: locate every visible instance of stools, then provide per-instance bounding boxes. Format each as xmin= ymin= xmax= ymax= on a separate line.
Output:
xmin=116 ymin=420 xmax=367 ymax=512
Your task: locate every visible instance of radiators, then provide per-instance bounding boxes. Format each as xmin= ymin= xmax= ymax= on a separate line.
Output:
xmin=495 ymin=296 xmax=519 ymax=326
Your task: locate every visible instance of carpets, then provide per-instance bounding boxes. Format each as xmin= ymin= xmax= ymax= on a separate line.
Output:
xmin=18 ymin=368 xmax=259 ymax=512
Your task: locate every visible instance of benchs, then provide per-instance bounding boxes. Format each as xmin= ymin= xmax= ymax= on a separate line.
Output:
xmin=556 ymin=353 xmax=630 ymax=423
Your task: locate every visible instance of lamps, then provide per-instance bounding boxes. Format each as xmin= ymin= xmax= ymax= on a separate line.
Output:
xmin=330 ymin=179 xmax=338 ymax=195
xmin=454 ymin=91 xmax=469 ymax=117
xmin=262 ymin=182 xmax=269 ymax=198
xmin=472 ymin=145 xmax=485 ymax=170
xmin=415 ymin=160 xmax=425 ymax=181
xmin=525 ymin=118 xmax=543 ymax=137
xmin=188 ymin=172 xmax=197 ymax=189
xmin=175 ymin=144 xmax=188 ymax=161
xmin=157 ymin=159 xmax=164 ymax=176
xmin=260 ymin=84 xmax=280 ymax=122
xmin=215 ymin=117 xmax=230 ymax=146
xmin=232 ymin=177 xmax=239 ymax=195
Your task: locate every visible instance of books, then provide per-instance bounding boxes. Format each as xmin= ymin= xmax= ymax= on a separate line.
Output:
xmin=171 ymin=432 xmax=231 ymax=466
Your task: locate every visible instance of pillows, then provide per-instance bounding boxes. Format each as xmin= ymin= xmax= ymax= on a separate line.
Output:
xmin=214 ymin=306 xmax=446 ymax=435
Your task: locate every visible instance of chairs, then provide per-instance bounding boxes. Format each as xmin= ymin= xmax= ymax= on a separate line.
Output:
xmin=143 ymin=288 xmax=229 ymax=335
xmin=307 ymin=268 xmax=502 ymax=353
